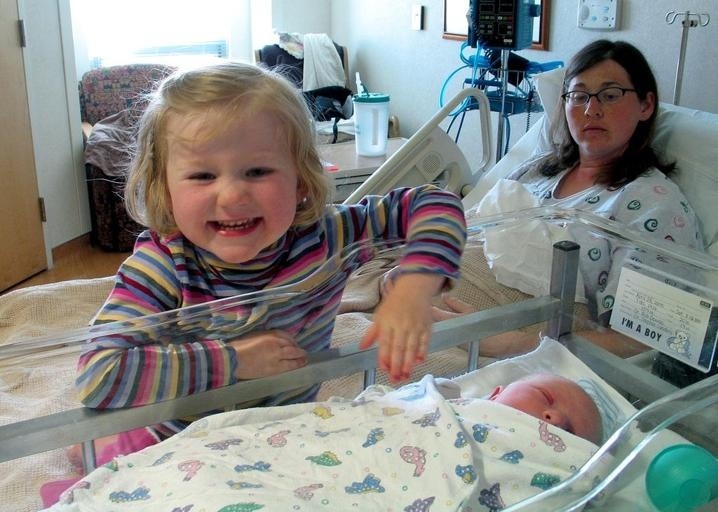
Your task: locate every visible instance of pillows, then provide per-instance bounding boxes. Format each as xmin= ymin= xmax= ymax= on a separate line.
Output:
xmin=533 ymin=65 xmax=718 ymax=249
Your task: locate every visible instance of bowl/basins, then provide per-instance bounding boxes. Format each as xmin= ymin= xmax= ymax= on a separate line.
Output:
xmin=646 ymin=443 xmax=717 ymax=512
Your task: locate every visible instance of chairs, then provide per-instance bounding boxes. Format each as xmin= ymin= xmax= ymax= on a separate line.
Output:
xmin=77 ymin=63 xmax=182 ymax=253
xmin=254 ymin=40 xmax=400 ymax=145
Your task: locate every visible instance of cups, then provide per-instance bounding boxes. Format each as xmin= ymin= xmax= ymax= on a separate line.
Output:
xmin=352 ymin=83 xmax=389 ymax=157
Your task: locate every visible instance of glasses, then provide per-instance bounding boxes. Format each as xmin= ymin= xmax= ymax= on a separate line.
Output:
xmin=560 ymin=86 xmax=636 ymax=108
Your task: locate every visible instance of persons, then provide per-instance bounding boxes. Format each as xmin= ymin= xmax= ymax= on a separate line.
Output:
xmin=489 ymin=374 xmax=603 ymax=447
xmin=67 ymin=54 xmax=467 ymax=473
xmin=377 ymin=38 xmax=705 ymax=359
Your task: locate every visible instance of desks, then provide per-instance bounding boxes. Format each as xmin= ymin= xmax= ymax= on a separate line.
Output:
xmin=315 ymin=137 xmax=408 ymax=203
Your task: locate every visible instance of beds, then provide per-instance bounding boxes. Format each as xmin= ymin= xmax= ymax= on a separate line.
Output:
xmin=0 ymin=89 xmax=718 ymax=512
xmin=0 ymin=206 xmax=718 ymax=512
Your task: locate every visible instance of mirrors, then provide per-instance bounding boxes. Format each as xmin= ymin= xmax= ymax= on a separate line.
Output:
xmin=441 ymin=0 xmax=550 ymax=51
